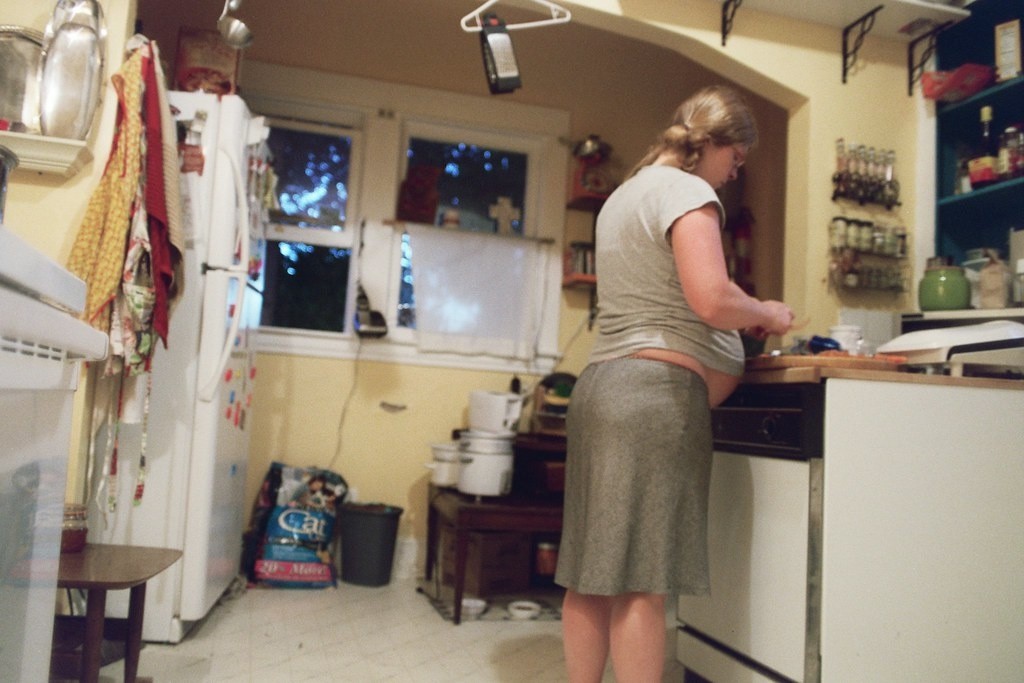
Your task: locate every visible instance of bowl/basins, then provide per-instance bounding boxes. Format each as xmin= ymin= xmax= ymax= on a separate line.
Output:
xmin=510 ymin=602 xmax=541 ymax=621
xmin=460 ymin=599 xmax=486 ymax=618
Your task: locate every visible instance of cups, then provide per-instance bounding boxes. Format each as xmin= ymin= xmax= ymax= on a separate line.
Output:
xmin=827 ymin=324 xmax=866 ymax=357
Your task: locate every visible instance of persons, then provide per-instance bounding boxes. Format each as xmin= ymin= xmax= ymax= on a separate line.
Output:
xmin=553 ymin=83 xmax=795 ymax=682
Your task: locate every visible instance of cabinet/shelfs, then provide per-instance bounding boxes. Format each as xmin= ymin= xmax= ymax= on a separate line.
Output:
xmin=670 ymin=378 xmax=1023 ymax=683
xmin=0 ymin=278 xmax=108 ymax=683
xmin=903 ymin=0 xmax=1024 ymax=318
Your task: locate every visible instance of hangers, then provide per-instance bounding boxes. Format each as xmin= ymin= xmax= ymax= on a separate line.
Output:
xmin=461 ymin=0 xmax=571 ymax=31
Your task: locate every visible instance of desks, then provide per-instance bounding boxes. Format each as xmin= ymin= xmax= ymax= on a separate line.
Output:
xmin=424 ymin=488 xmax=563 ymax=625
xmin=58 ymin=543 xmax=184 ymax=683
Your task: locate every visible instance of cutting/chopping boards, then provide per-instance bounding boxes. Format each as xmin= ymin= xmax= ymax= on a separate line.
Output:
xmin=745 ymin=354 xmax=902 ymax=371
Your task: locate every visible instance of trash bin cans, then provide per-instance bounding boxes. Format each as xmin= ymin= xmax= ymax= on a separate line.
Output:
xmin=340 ymin=503 xmax=404 ymax=588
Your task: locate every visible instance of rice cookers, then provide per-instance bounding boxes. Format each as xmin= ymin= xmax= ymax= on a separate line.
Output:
xmin=424 ymin=428 xmax=516 ymax=499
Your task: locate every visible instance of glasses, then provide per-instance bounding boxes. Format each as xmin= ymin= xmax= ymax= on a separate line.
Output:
xmin=729 ymin=144 xmax=747 ymax=167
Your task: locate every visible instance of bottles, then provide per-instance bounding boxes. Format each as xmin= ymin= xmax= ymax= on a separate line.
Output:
xmin=60 ymin=502 xmax=89 ymax=555
xmin=834 ymin=137 xmax=896 ymax=198
xmin=563 ymin=240 xmax=595 ymax=276
xmin=951 ymin=106 xmax=1024 ymax=194
xmin=919 ymin=266 xmax=968 ymax=310
xmin=828 ymin=216 xmax=907 ymax=290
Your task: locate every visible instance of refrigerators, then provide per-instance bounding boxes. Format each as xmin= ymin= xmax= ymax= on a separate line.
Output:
xmin=55 ymin=73 xmax=279 ymax=641
